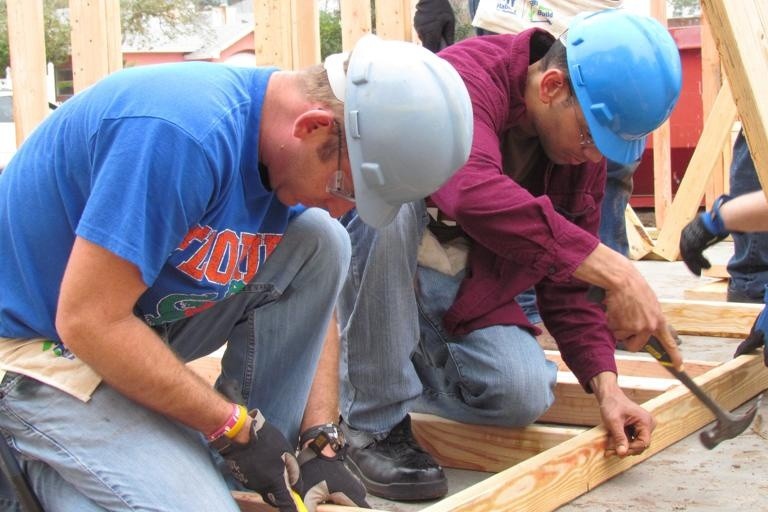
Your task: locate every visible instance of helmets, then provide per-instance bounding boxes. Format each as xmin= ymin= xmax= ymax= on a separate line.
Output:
xmin=344 ymin=34 xmax=475 ymax=230
xmin=565 ymin=5 xmax=684 ymax=165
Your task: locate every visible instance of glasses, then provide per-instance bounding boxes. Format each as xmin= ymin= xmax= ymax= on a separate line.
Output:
xmin=565 ymin=80 xmax=595 ymax=148
xmin=317 ymin=107 xmax=357 ymax=205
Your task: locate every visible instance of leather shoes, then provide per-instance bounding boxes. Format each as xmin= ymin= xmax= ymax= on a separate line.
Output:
xmin=347 ymin=414 xmax=448 ymax=500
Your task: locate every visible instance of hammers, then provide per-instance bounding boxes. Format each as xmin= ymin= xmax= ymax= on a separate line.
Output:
xmin=585 ymin=285 xmax=758 ymax=449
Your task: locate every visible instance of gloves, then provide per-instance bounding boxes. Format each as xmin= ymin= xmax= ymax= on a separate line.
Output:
xmin=293 ymin=422 xmax=370 ymax=511
xmin=207 ymin=409 xmax=301 ymax=512
xmin=733 ymin=285 xmax=768 ymax=366
xmin=680 ymin=195 xmax=735 ymax=276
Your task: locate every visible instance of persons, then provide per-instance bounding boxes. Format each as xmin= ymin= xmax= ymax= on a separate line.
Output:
xmin=721 ymin=114 xmax=767 ymax=303
xmin=334 ymin=4 xmax=688 ymax=503
xmin=411 ymin=0 xmax=684 ymax=354
xmin=0 ymin=26 xmax=474 ymax=512
xmin=678 ymin=189 xmax=767 ymax=278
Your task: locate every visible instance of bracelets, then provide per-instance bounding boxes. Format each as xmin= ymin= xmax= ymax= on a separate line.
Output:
xmin=224 ymin=405 xmax=252 ymax=441
xmin=202 ymin=402 xmax=240 ymax=438
